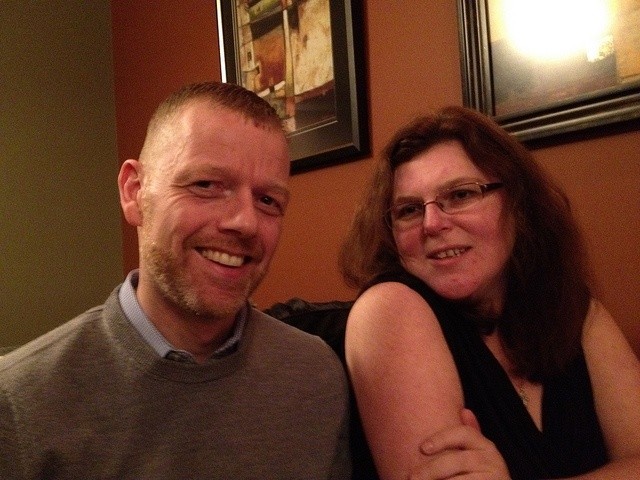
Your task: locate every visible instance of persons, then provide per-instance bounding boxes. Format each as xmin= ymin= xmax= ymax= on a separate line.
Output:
xmin=0 ymin=80 xmax=355 ymax=480
xmin=338 ymin=103 xmax=639 ymax=480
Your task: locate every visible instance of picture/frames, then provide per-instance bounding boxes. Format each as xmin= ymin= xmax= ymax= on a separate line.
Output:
xmin=217 ymin=0 xmax=373 ymax=176
xmin=455 ymin=0 xmax=640 ymax=152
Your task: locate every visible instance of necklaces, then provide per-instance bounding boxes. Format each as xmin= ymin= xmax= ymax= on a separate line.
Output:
xmin=520 ymin=389 xmax=529 ymax=406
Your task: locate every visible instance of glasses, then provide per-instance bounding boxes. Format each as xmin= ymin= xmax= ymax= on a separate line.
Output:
xmin=383 ymin=182 xmax=505 ymax=232
